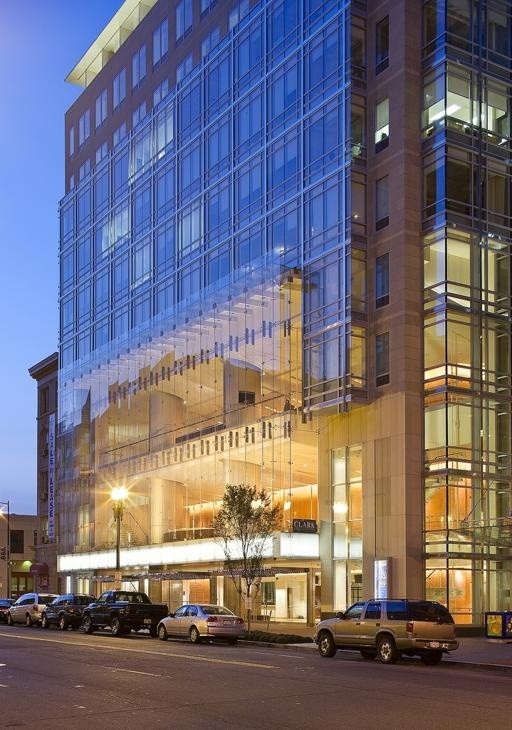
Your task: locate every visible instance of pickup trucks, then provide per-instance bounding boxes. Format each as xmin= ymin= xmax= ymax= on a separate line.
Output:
xmin=81 ymin=589 xmax=169 ymax=637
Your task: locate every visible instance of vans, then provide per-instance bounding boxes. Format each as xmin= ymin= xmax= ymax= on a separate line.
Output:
xmin=6 ymin=592 xmax=61 ymax=627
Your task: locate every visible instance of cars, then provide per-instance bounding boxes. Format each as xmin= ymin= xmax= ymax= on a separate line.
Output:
xmin=157 ymin=603 xmax=246 ymax=646
xmin=1 ymin=599 xmax=18 ymax=623
xmin=42 ymin=594 xmax=95 ymax=630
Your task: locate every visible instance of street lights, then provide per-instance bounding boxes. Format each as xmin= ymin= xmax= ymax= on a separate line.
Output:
xmin=0 ymin=501 xmax=11 ymax=598
xmin=112 ymin=484 xmax=130 ymax=591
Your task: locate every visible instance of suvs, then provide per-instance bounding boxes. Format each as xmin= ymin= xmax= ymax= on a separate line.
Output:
xmin=314 ymin=598 xmax=459 ymax=663
